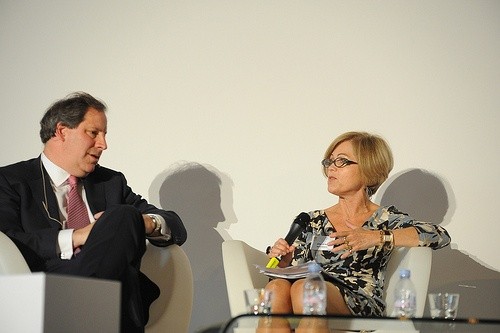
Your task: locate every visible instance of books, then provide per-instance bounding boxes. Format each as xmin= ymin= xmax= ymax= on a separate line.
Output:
xmin=255 ymin=261 xmax=357 ymax=290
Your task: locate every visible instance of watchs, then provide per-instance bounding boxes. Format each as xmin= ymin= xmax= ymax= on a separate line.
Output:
xmin=147 ymin=216 xmax=162 ymax=237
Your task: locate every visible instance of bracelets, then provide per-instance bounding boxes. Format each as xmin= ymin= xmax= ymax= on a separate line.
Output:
xmin=378 ymin=230 xmax=384 ymax=249
xmin=381 ymin=230 xmax=395 ymax=252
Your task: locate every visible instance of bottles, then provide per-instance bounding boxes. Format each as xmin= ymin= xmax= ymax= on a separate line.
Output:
xmin=394 ymin=270 xmax=416 ymax=318
xmin=303 ymin=263 xmax=327 ymax=315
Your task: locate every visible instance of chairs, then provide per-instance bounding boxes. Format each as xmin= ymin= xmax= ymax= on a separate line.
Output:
xmin=221 ymin=241 xmax=432 ymax=333
xmin=0 ymin=230 xmax=194 ymax=333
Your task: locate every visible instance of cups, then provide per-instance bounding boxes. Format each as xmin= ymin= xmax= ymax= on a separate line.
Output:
xmin=242 ymin=288 xmax=273 ymax=315
xmin=428 ymin=292 xmax=460 ymax=320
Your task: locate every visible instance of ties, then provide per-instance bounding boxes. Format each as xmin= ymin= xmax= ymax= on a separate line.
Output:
xmin=64 ymin=175 xmax=91 ymax=256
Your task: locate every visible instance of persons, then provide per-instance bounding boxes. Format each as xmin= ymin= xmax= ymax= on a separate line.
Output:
xmin=1 ymin=90 xmax=188 ymax=333
xmin=255 ymin=132 xmax=453 ymax=333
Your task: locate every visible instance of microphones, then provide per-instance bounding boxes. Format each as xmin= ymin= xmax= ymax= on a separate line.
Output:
xmin=266 ymin=212 xmax=310 ymax=269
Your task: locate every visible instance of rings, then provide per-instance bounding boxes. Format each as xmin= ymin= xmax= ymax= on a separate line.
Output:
xmin=343 ymin=237 xmax=348 ymax=244
xmin=346 ymin=244 xmax=351 ymax=250
xmin=349 ymin=250 xmax=352 ymax=254
xmin=265 ymin=246 xmax=273 ymax=254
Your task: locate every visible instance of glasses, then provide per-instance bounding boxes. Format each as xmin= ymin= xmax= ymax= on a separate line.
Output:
xmin=321 ymin=157 xmax=359 ymax=169
